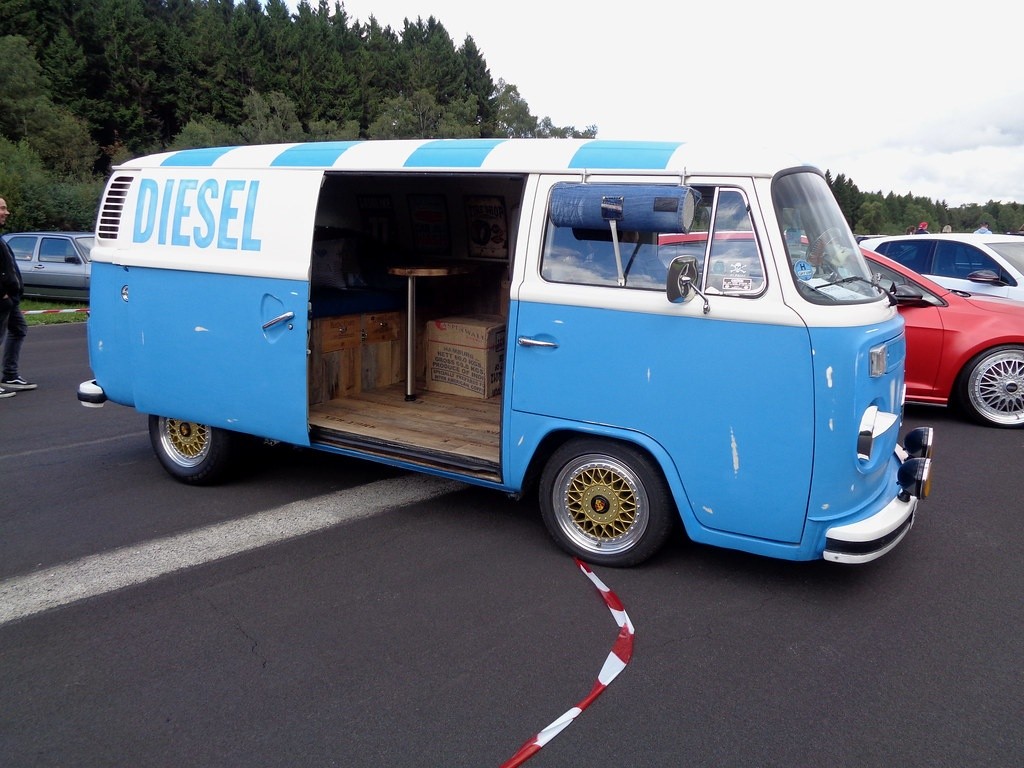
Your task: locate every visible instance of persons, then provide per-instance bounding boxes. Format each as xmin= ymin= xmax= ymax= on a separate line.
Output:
xmin=0 ymin=194 xmax=38 ymax=398
xmin=973 ymin=221 xmax=993 ymax=234
xmin=906 ymin=221 xmax=930 ymax=235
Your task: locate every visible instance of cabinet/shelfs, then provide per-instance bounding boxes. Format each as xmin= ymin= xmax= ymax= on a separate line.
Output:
xmin=310 ymin=311 xmax=407 ymax=404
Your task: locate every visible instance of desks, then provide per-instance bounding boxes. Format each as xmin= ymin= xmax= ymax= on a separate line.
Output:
xmin=389 ymin=265 xmax=470 ymax=402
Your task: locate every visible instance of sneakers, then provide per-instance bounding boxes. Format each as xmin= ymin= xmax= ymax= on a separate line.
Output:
xmin=0 ymin=387 xmax=16 ymax=398
xmin=0 ymin=375 xmax=37 ymax=389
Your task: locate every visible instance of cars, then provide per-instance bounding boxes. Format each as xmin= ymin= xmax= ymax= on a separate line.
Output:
xmin=612 ymin=229 xmax=1024 ymax=430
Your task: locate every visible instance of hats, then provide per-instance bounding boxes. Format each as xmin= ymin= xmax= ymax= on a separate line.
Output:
xmin=918 ymin=222 xmax=927 ymax=229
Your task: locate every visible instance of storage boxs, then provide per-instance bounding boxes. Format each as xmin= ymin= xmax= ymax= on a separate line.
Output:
xmin=425 ymin=318 xmax=506 ymax=400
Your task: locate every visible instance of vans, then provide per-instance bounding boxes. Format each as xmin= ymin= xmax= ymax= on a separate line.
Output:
xmin=1 ymin=231 xmax=96 ymax=302
xmin=79 ymin=135 xmax=935 ymax=568
